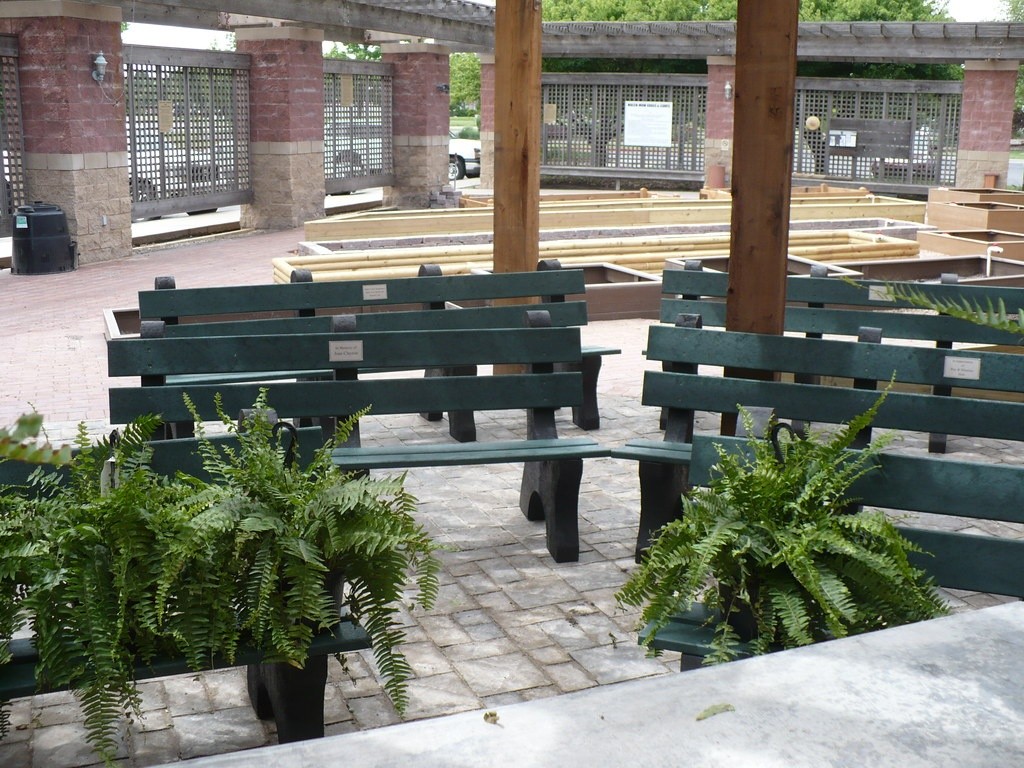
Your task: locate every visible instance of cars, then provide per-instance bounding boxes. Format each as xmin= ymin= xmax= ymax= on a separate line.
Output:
xmin=3 ymin=118 xmax=222 ymax=223
xmin=872 ymin=125 xmax=939 ymax=185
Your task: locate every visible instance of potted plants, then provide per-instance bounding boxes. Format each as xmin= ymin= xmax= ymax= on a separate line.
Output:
xmin=1 ymin=402 xmax=453 ymax=768
xmin=614 ymin=370 xmax=960 ymax=666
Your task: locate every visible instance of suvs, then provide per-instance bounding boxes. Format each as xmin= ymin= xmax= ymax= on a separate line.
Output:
xmin=324 ymin=118 xmax=481 ymax=180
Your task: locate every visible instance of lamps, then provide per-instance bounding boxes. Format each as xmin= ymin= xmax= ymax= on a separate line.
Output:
xmin=93 ymin=51 xmax=108 ymax=82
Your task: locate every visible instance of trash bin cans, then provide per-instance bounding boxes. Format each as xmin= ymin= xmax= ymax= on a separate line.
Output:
xmin=11 ymin=204 xmax=73 ymax=275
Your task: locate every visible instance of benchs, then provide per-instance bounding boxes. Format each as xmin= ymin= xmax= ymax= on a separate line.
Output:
xmin=638 ymin=437 xmax=1023 ymax=674
xmin=111 ymin=259 xmax=1024 ymax=563
xmin=0 ymin=429 xmax=373 ymax=745
